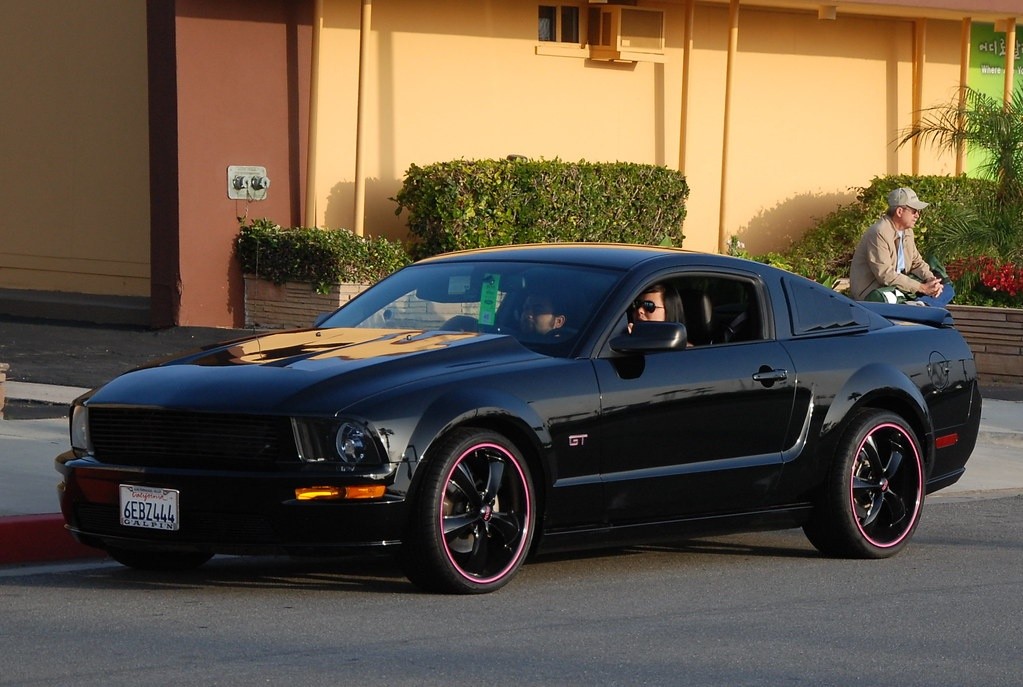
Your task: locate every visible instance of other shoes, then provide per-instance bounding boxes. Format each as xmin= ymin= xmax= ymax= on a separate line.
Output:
xmin=902 ymin=301 xmax=925 ymax=307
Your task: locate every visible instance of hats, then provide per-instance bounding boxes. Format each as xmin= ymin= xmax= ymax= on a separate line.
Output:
xmin=888 ymin=188 xmax=929 ymax=210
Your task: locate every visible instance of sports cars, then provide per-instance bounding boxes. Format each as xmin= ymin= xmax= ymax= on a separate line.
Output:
xmin=47 ymin=239 xmax=988 ymax=599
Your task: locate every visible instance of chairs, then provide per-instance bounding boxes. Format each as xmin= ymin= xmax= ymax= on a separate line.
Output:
xmin=552 ymin=270 xmax=588 ymax=332
xmin=682 ymin=290 xmax=727 ymax=347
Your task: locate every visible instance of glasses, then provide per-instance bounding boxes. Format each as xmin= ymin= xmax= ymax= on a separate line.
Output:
xmin=632 ymin=299 xmax=665 ymax=312
xmin=902 ymin=207 xmax=923 ymax=215
xmin=524 ymin=305 xmax=565 ymax=317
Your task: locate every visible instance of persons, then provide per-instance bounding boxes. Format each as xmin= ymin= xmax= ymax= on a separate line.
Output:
xmin=628 ymin=284 xmax=694 ymax=348
xmin=520 ymin=292 xmax=569 ymax=338
xmin=850 ymin=187 xmax=955 ymax=308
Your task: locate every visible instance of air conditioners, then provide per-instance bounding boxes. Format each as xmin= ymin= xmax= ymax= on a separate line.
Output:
xmin=588 ymin=3 xmax=665 ymax=54
